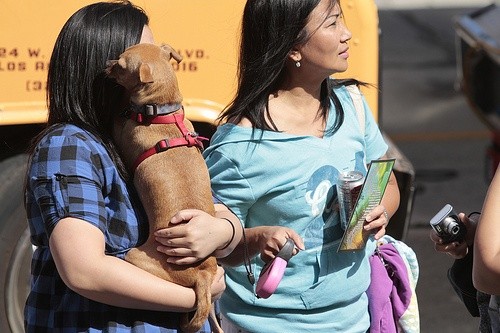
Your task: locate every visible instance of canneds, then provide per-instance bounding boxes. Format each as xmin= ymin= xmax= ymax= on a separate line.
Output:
xmin=337 ymin=171 xmax=365 ymax=230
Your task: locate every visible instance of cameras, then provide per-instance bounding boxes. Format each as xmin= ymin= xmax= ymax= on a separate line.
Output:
xmin=430 ymin=204 xmax=467 ymax=246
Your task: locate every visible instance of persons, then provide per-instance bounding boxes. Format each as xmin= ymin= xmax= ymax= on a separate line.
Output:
xmin=430 ymin=162 xmax=500 ymax=333
xmin=202 ymin=0 xmax=400 ymax=333
xmin=24 ymin=0 xmax=243 ymax=333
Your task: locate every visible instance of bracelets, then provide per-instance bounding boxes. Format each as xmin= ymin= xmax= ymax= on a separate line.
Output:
xmin=382 ymin=206 xmax=389 ymax=223
xmin=467 ymin=211 xmax=481 ymax=219
xmin=216 ymin=218 xmax=235 ymax=250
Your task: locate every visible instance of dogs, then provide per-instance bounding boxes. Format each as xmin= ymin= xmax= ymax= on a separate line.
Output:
xmin=105 ymin=42 xmax=223 ymax=333
